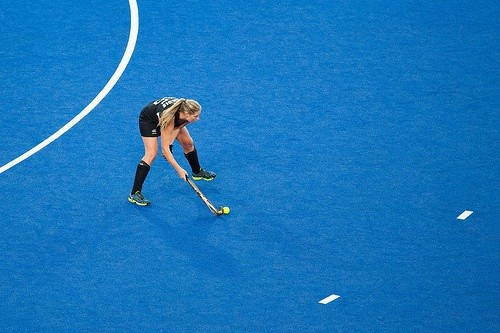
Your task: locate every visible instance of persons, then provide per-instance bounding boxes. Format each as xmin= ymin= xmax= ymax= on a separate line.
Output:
xmin=128 ymin=97 xmax=216 ymax=206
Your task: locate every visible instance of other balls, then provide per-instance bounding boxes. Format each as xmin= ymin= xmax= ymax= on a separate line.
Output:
xmin=223 ymin=207 xmax=230 ymax=214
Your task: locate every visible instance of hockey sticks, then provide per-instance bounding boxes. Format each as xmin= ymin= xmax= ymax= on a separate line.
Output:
xmin=185 ymin=175 xmax=223 ymax=215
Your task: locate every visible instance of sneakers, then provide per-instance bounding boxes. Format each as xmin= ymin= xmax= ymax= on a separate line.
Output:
xmin=128 ymin=191 xmax=150 ymax=206
xmin=192 ymin=168 xmax=216 ymax=181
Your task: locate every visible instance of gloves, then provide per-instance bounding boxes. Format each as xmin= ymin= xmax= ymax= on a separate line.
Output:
xmin=162 ymin=145 xmax=173 ymax=161
